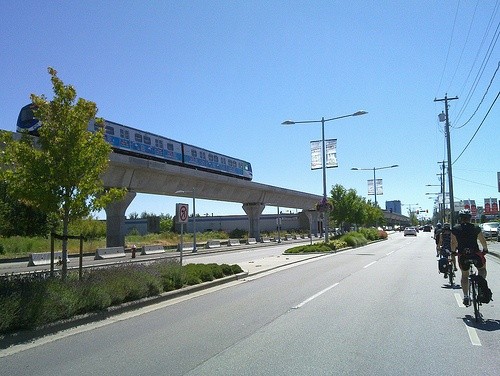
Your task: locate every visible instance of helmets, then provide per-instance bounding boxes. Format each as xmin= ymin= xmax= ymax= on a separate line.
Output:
xmin=443 ymin=223 xmax=450 ymax=228
xmin=457 ymin=209 xmax=472 ymax=217
xmin=437 ymin=223 xmax=442 ymax=226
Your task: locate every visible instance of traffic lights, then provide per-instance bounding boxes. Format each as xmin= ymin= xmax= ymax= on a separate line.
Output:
xmin=416 ymin=209 xmax=419 ymax=214
xmin=425 ymin=209 xmax=428 ymax=213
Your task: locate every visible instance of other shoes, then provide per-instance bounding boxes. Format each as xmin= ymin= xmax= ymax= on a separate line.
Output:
xmin=454 ymin=267 xmax=458 ymax=271
xmin=436 ymin=254 xmax=439 ymax=257
xmin=463 ymin=297 xmax=469 ymax=305
xmin=443 ymin=274 xmax=448 ymax=278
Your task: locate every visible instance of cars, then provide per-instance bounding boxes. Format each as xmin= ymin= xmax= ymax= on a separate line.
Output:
xmin=404 ymin=227 xmax=417 ymax=236
xmin=480 ymin=222 xmax=500 ymax=242
xmin=423 ymin=225 xmax=431 ymax=232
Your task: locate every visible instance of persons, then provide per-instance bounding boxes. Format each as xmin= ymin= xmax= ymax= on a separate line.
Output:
xmin=451 ymin=209 xmax=488 ymax=305
xmin=433 ymin=222 xmax=457 ymax=278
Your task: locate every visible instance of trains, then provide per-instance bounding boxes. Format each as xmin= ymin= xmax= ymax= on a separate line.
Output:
xmin=17 ymin=102 xmax=253 ymax=181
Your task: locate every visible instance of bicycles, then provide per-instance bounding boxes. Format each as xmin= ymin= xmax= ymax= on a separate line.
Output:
xmin=452 ymin=250 xmax=488 ymax=322
xmin=431 ymin=235 xmax=454 ymax=286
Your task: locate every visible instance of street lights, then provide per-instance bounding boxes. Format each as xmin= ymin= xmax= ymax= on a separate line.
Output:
xmin=281 ymin=110 xmax=368 ymax=243
xmin=350 ymin=164 xmax=399 ymax=229
xmin=402 ymin=202 xmax=422 ymax=227
